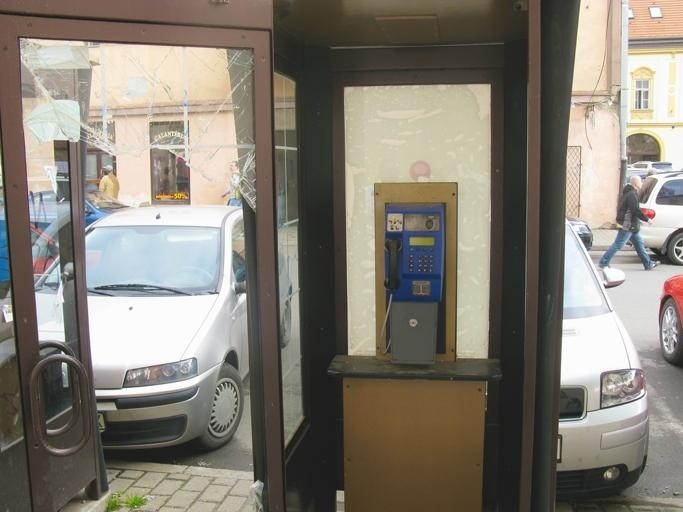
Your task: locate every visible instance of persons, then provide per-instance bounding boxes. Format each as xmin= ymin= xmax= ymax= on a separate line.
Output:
xmin=648 ymin=168 xmax=656 ymax=175
xmin=98 ymin=165 xmax=119 ymax=198
xmin=598 ymin=175 xmax=661 ymax=270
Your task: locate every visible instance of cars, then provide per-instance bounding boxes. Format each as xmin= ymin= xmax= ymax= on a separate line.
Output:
xmin=627 ymin=160 xmax=683 ymax=265
xmin=1 ymin=188 xmax=132 ymax=278
xmin=659 ymin=273 xmax=683 ymax=363
xmin=0 ymin=204 xmax=292 ymax=450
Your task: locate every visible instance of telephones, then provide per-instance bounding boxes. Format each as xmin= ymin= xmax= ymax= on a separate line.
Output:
xmin=383 ymin=203 xmax=445 ymax=303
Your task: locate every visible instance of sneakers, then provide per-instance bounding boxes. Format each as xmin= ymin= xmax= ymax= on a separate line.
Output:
xmin=597 ymin=264 xmax=610 ymax=271
xmin=645 ymin=260 xmax=660 ymax=270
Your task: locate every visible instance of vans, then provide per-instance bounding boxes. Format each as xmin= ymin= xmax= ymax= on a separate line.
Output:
xmin=557 ymin=217 xmax=650 ymax=502
